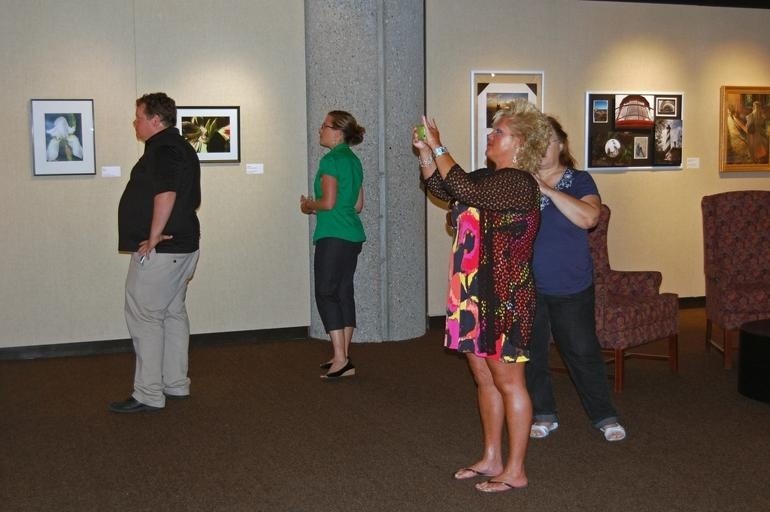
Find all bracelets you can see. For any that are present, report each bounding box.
[434,146,450,159]
[417,157,435,169]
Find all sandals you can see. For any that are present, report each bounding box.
[600,420,626,442]
[530,421,558,438]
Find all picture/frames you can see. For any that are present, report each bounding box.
[471,69,545,171]
[30,98,97,176]
[584,90,684,173]
[718,85,770,173]
[174,105,241,163]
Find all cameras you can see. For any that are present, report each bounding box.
[416,124,426,140]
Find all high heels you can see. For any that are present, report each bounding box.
[320,359,355,379]
[320,355,351,369]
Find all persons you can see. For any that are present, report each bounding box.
[108,91,202,412]
[412,98,554,492]
[525,112,627,441]
[300,109,367,380]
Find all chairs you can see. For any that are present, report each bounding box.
[701,189,769,371]
[587,203,681,393]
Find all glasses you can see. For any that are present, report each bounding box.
[491,128,514,137]
[321,124,332,129]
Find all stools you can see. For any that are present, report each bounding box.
[738,321,768,400]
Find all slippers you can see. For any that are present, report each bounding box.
[455,467,500,479]
[476,479,528,493]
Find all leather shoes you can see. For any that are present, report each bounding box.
[164,392,189,400]
[108,397,165,412]
